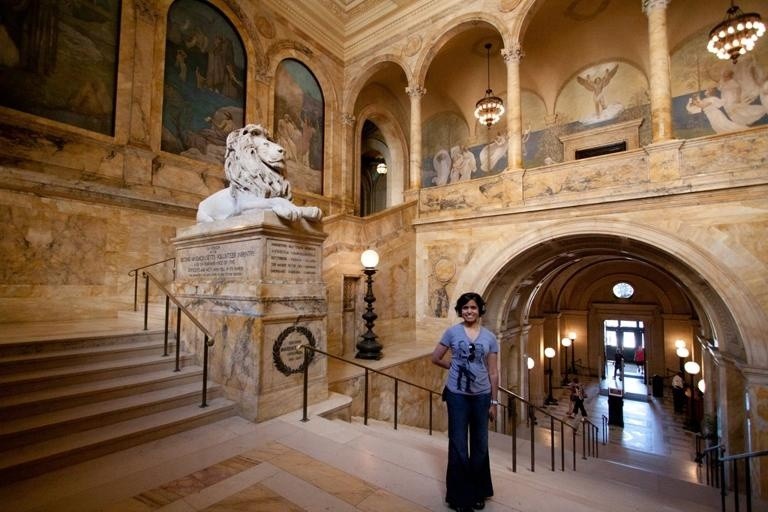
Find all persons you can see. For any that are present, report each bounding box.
[565,383,574,415]
[633,346,644,374]
[692,65,768,135]
[576,63,619,120]
[431,291,500,512]
[431,123,533,186]
[174,18,243,154]
[568,377,588,422]
[614,348,624,381]
[276,111,312,164]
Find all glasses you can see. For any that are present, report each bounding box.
[468,343,475,361]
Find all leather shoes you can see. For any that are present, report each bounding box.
[449,498,485,512]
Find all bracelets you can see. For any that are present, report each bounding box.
[490,399,498,406]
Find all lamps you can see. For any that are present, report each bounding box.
[376,162,388,174]
[704,0,766,66]
[475,42,504,130]
